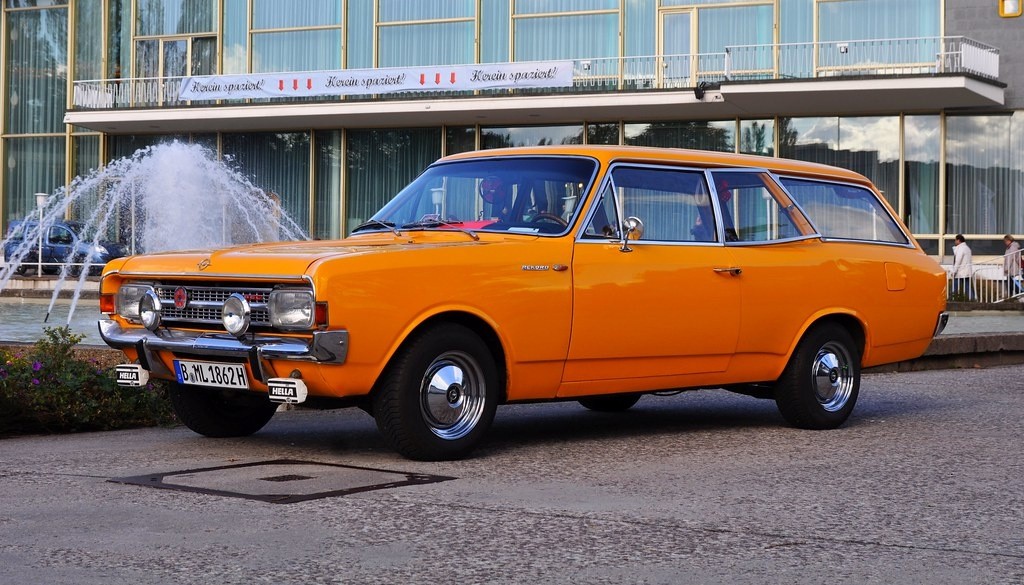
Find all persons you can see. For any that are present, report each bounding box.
[1003,234,1024,297]
[951,234,974,302]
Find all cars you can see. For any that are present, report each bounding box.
[98,146,949,461]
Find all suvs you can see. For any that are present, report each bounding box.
[3,220,123,277]
